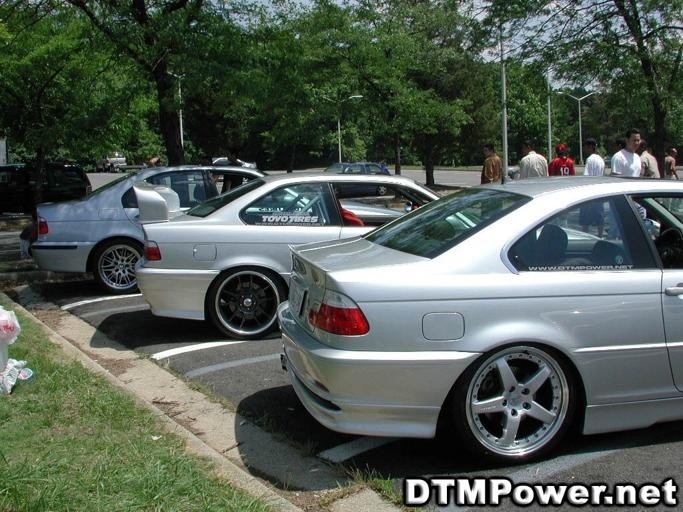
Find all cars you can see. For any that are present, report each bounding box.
[20,165,269,293]
[322,161,390,176]
[134,173,601,342]
[276,175,683,461]
[191,157,258,181]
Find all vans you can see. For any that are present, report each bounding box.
[603,156,612,168]
[0,164,92,213]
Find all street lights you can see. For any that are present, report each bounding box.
[166,71,186,150]
[556,90,602,167]
[318,93,369,163]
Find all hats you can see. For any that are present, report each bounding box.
[583,139,596,145]
[556,144,567,152]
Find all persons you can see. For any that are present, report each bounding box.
[220,144,244,195]
[664,147,678,180]
[609,127,645,237]
[146,156,167,186]
[478,140,661,216]
[194,153,219,201]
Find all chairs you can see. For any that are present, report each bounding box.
[593,241,629,265]
[535,225,593,267]
[194,183,219,202]
[336,197,365,226]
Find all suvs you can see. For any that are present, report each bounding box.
[96,152,127,172]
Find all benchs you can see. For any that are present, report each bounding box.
[117,165,144,172]
